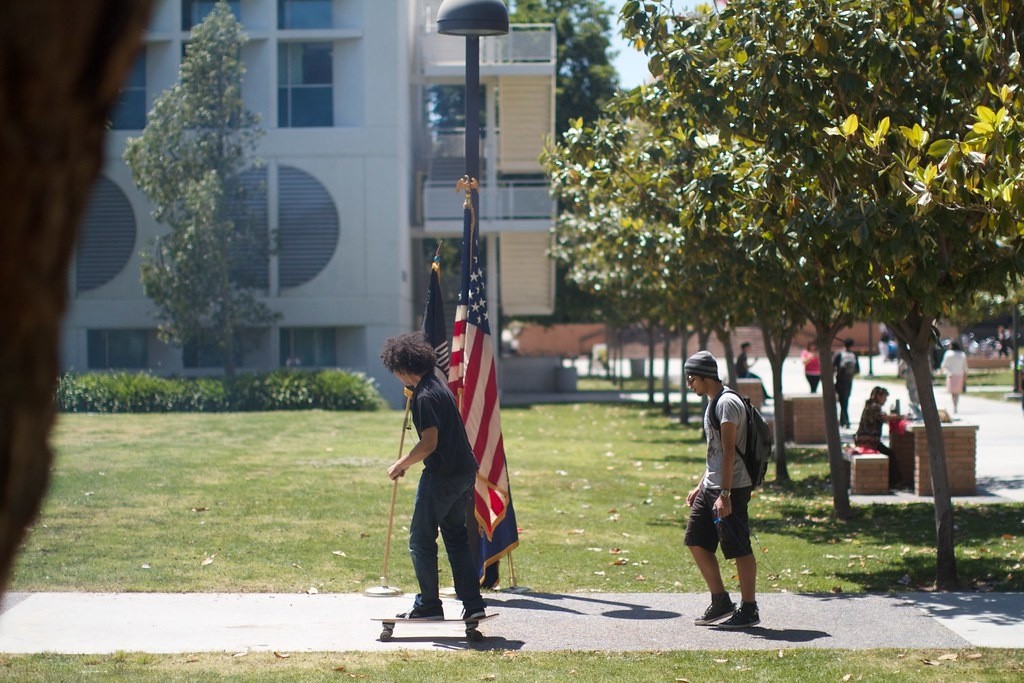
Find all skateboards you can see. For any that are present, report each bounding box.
[363,611,501,642]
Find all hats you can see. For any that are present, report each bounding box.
[685,351,719,378]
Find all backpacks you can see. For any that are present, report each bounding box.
[839,350,856,376]
[711,390,774,486]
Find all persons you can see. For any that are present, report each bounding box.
[683,349,772,629]
[736,342,772,399]
[832,337,860,429]
[898,350,934,406]
[380,330,488,621]
[940,339,968,413]
[801,341,821,393]
[857,386,903,488]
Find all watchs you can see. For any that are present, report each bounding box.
[719,488,732,498]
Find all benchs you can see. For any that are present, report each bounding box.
[841,444,889,495]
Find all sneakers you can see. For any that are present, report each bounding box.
[394,605,445,620]
[694,601,738,625]
[719,607,761,629]
[461,598,488,620]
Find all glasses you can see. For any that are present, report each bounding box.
[684,375,697,386]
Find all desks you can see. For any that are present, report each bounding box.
[722,377,763,413]
[783,392,839,445]
[886,415,980,497]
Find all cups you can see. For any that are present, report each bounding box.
[712,507,734,542]
[890,410,895,413]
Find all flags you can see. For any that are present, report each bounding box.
[403,254,450,395]
[446,186,520,586]
